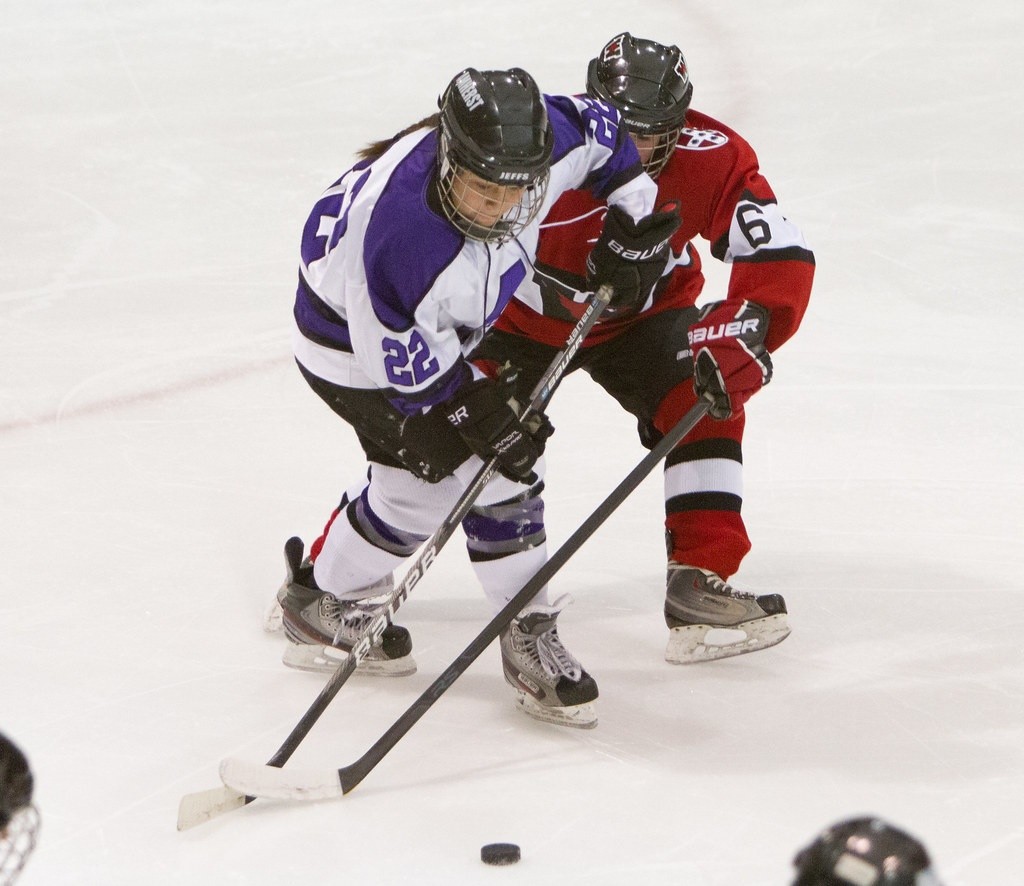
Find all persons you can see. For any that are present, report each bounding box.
[278,66,683,730]
[282,31,816,666]
[0,731,41,886]
[790,817,940,886]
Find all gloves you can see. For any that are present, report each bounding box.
[687,299,773,421]
[443,366,555,487]
[586,201,683,319]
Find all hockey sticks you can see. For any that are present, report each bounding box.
[215,396,715,805]
[144,284,616,834]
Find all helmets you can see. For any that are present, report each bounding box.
[438,67,552,247]
[791,818,930,886]
[586,32,693,180]
[1,737,41,886]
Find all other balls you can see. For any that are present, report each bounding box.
[480,843,521,867]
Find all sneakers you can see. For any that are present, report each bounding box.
[277,537,418,678]
[501,594,599,730]
[663,525,791,666]
